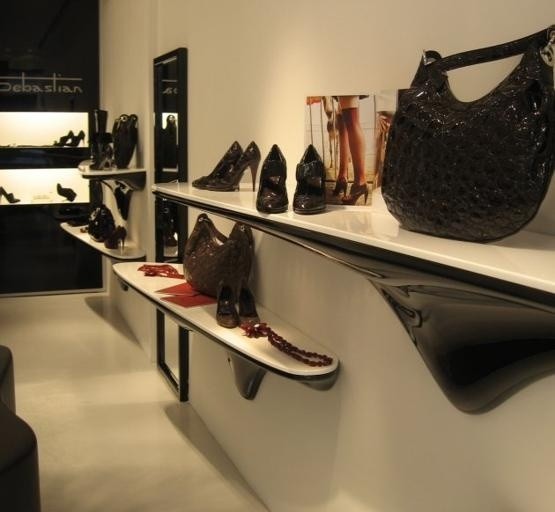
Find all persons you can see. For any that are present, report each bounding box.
[332,96,369,206]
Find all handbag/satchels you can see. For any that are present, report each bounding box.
[382,24,555,243]
[183,214,252,298]
[89,204,114,241]
[112,114,137,168]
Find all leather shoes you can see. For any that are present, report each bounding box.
[57,184,76,201]
[216,278,260,330]
[257,144,288,212]
[294,144,326,214]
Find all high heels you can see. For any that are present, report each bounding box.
[54,130,84,146]
[192,141,260,191]
[105,225,126,249]
[90,146,111,169]
[333,180,368,203]
[0,187,20,202]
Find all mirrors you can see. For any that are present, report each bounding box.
[152,46,190,403]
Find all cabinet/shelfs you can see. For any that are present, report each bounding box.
[59,220,147,294]
[149,177,472,415]
[110,261,341,402]
[77,159,147,193]
[0,92,103,290]
